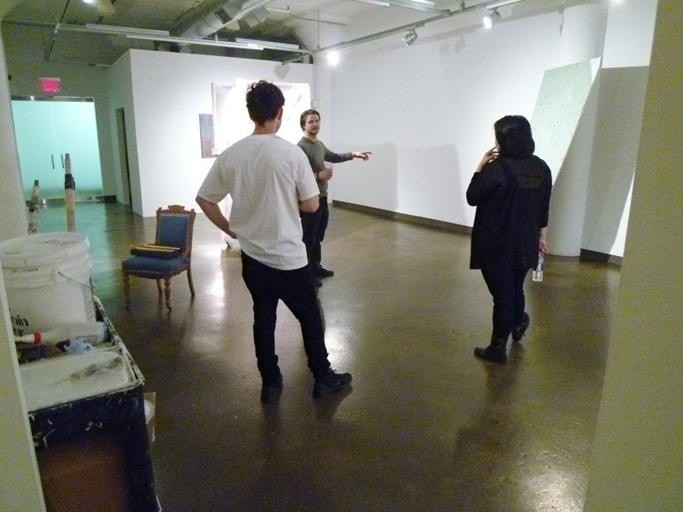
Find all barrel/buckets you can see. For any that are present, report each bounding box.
[0,233,96,336]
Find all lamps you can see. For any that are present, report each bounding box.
[54,21,319,53]
[400,6,508,49]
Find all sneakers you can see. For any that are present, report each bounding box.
[312,373,352,400]
[260,375,283,402]
[512,312,530,343]
[475,346,508,365]
[313,265,333,288]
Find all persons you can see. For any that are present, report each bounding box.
[295,109,373,288]
[194,80,352,404]
[466,114,553,365]
[32,180,40,205]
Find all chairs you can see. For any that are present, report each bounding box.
[120,202,200,314]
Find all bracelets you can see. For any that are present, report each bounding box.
[317,169,322,181]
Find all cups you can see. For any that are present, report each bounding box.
[324,163,333,170]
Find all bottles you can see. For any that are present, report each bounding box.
[531,251,543,283]
[12,320,107,352]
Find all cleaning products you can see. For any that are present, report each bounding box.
[13,322,104,347]
